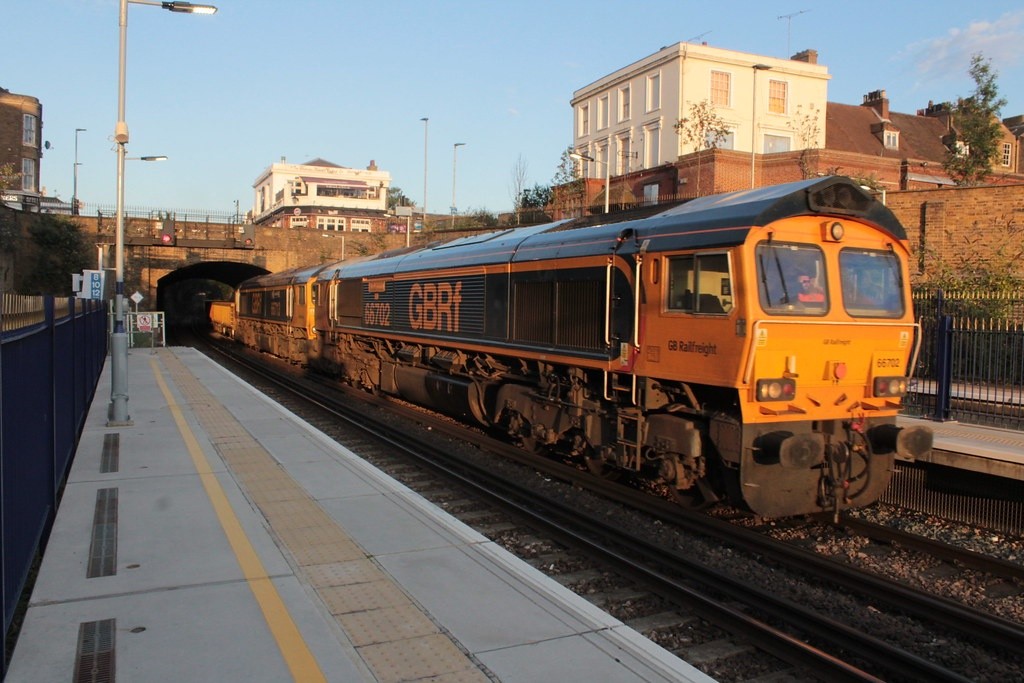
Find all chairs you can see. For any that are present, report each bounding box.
[687,293,726,313]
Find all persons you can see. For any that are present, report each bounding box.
[798,274,825,302]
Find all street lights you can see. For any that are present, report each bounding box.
[73,128,87,199]
[421,117,428,219]
[451,143,467,227]
[111,0,217,425]
[569,152,610,214]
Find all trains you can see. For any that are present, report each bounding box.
[206,174,924,525]
